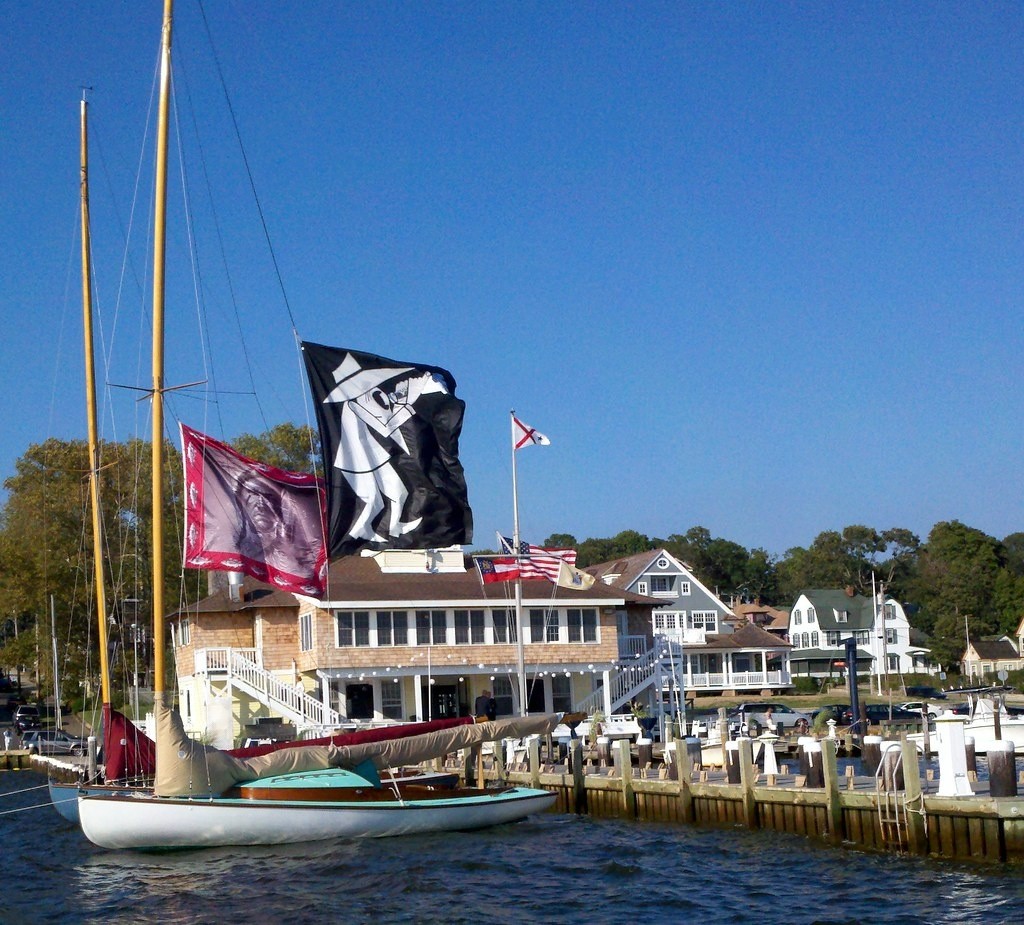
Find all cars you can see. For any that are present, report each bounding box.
[19,730,88,756]
[842,703,922,726]
[13,705,39,726]
[738,703,814,730]
[906,687,947,699]
[895,701,943,719]
[950,703,970,715]
[806,704,851,724]
[14,715,42,736]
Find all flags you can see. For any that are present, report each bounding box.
[558,560,595,590]
[178,421,328,607]
[513,416,551,449]
[476,557,520,585]
[500,536,578,585]
[299,340,473,566]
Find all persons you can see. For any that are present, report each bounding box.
[765,708,773,731]
[475,690,497,721]
[3,728,13,751]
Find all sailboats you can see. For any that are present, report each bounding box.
[74,1,588,851]
[47,88,491,824]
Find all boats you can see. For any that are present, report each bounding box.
[907,685,1024,754]
[666,719,765,768]
